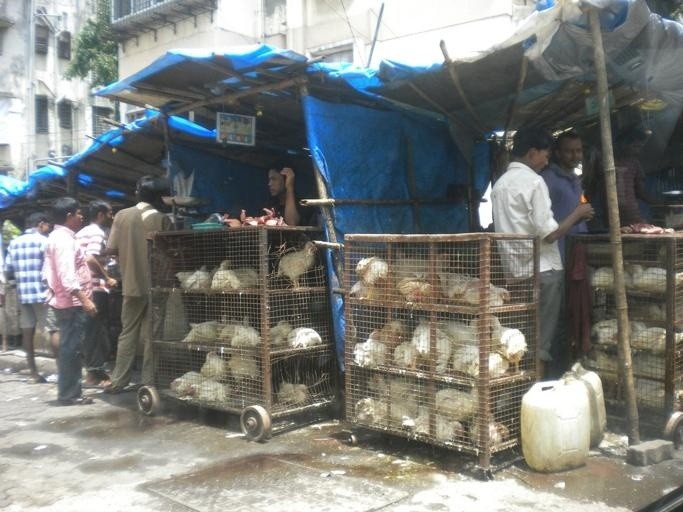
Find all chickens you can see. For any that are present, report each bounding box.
[585,259,683,413]
[168,240,323,405]
[348,252,529,449]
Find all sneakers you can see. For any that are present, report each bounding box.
[24,369,125,406]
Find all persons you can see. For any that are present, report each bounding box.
[490,127,594,379]
[262,161,317,226]
[540,130,591,351]
[0,173,164,406]
[597,123,661,228]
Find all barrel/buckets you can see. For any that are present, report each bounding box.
[521,373,591,473]
[569,362,607,448]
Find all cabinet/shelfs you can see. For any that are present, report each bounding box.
[344,232,539,465]
[579,231,683,424]
[138,227,335,418]
